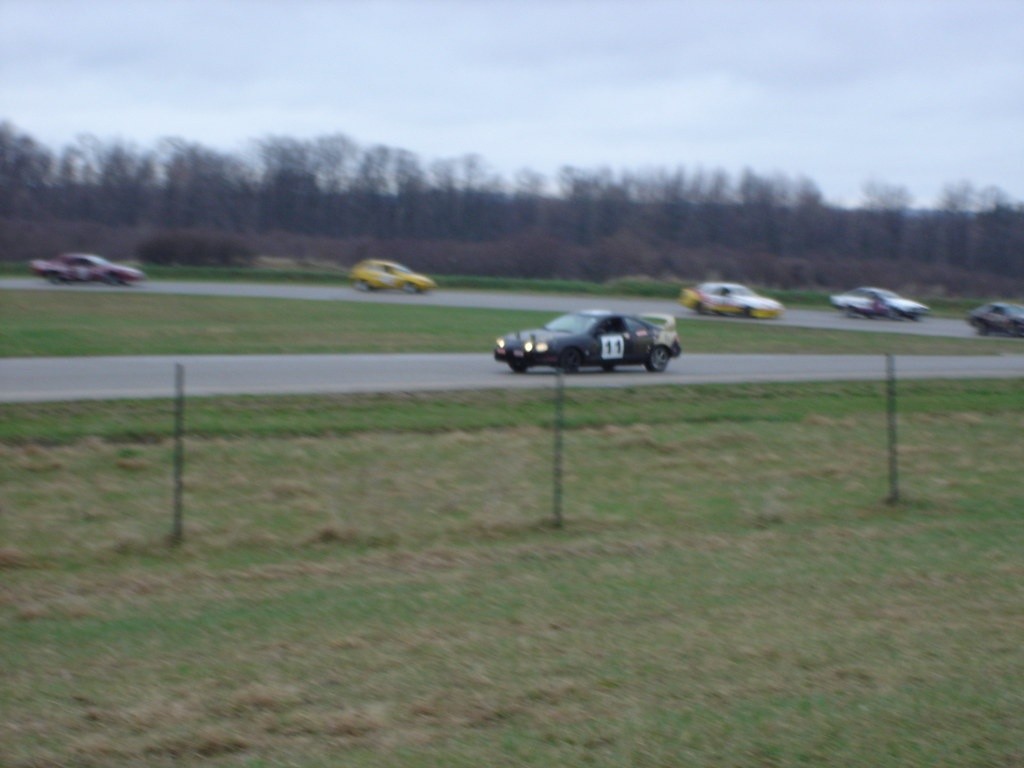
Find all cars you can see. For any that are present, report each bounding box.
[31,252,144,287]
[495,307,682,373]
[345,259,438,294]
[830,285,932,321]
[965,301,1024,338]
[680,280,786,319]
